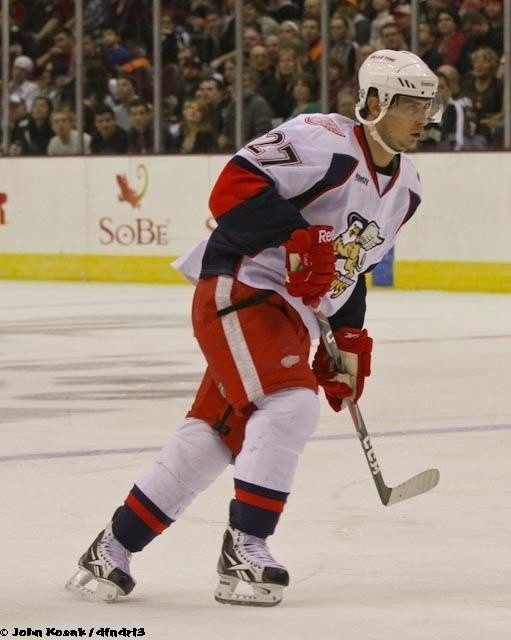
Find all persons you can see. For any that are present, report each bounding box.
[65,47,442,596]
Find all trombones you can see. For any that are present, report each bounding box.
[290,254,440,505]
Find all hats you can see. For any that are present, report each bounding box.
[14,55,34,73]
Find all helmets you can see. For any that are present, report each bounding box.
[355,49,439,125]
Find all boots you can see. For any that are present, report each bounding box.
[217,523,289,587]
[78,518,137,596]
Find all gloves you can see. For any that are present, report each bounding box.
[312,328,372,412]
[282,225,336,308]
